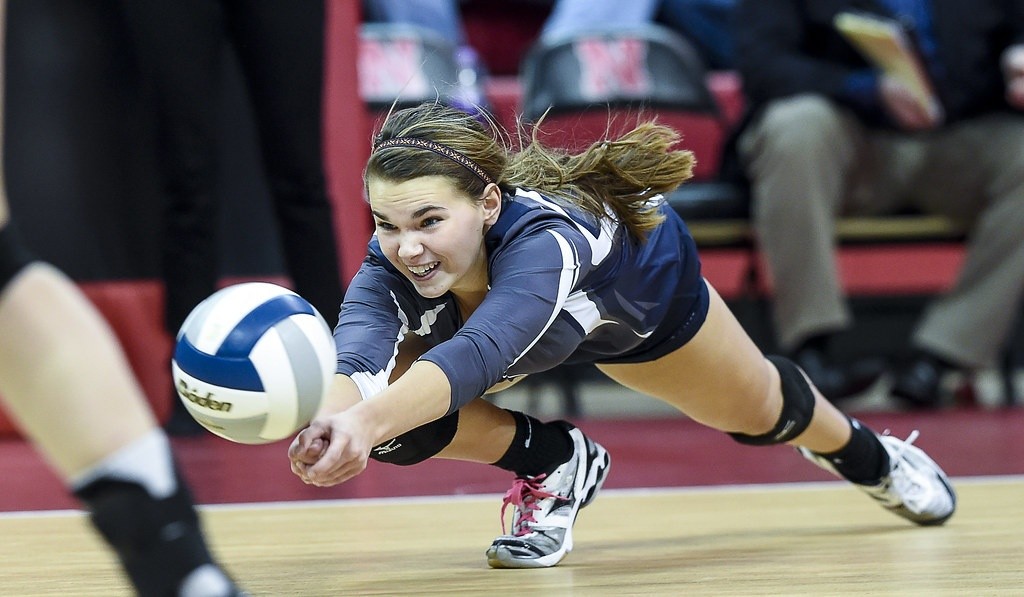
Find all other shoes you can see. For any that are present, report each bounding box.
[87,485,245,597]
[810,341,1004,415]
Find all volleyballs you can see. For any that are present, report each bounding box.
[172,282,337,445]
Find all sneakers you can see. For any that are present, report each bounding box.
[796,423,957,526]
[484,418,612,569]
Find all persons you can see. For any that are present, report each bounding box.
[289,101,957,568]
[0,188,243,597]
[726,0,1023,406]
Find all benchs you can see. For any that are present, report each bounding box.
[0,62,1024,439]
